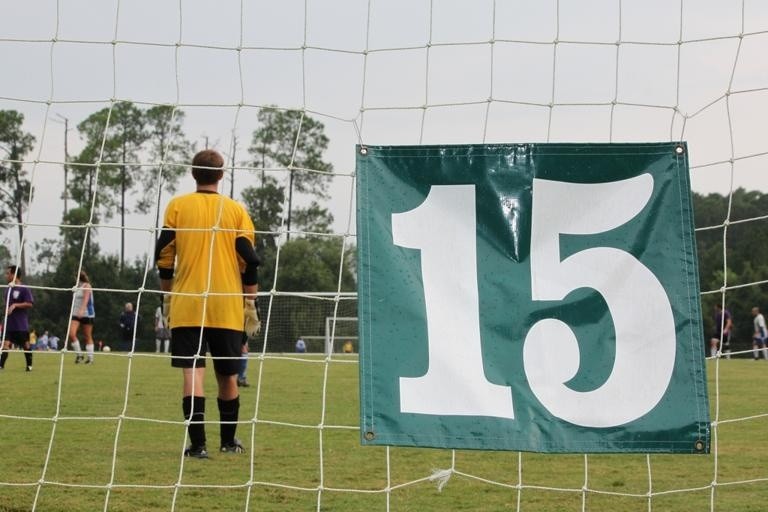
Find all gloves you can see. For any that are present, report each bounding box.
[244,297,262,341]
[159,291,172,340]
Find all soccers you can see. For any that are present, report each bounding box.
[102,345,112,352]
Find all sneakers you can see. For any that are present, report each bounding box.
[220,444,246,455]
[185,445,208,459]
[25,366,32,372]
[75,356,95,365]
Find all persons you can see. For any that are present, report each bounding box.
[340,340,353,353]
[118,302,143,353]
[48,335,60,350]
[153,148,262,460]
[41,330,49,351]
[36,335,45,351]
[234,332,261,388]
[152,304,171,355]
[294,336,306,353]
[0,263,36,371]
[68,270,96,366]
[29,328,37,350]
[710,301,733,361]
[749,305,768,362]
